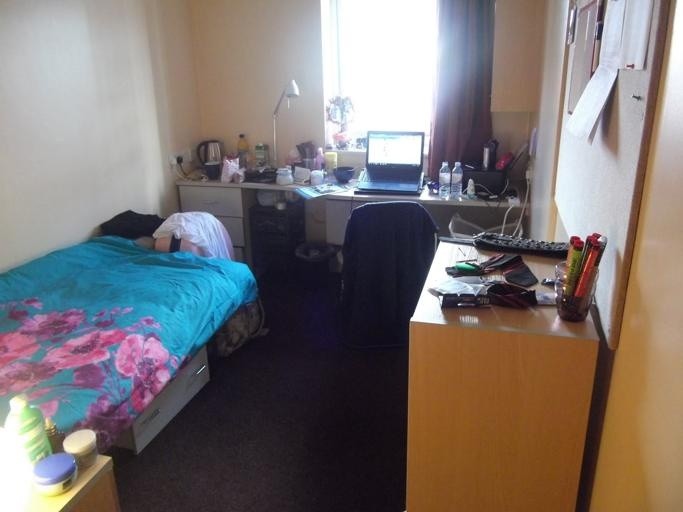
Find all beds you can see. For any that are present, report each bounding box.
[2,235,263,435]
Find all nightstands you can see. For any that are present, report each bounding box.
[34,453,123,512]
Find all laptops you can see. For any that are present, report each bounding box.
[358,131,425,193]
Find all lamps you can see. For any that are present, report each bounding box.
[273,79,301,163]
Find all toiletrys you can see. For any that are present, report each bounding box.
[45,422,62,452]
[62,429,99,468]
[34,452,79,495]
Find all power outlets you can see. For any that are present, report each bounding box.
[168,153,185,168]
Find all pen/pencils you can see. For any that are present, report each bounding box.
[560,234,608,310]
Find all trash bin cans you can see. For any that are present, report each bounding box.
[293,242,343,291]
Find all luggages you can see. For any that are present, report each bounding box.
[153,209,236,262]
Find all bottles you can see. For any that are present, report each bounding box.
[438,161,451,199]
[316,146,324,169]
[237,133,250,168]
[467,178,476,199]
[2,392,53,470]
[450,161,464,200]
[254,143,271,167]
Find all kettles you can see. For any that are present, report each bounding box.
[177,140,225,181]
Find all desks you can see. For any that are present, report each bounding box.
[175,164,530,272]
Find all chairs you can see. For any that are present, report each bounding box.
[339,201,440,353]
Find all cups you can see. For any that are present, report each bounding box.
[205,163,219,181]
[556,261,599,322]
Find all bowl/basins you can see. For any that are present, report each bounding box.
[334,166,355,183]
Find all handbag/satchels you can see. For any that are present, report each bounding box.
[215,292,265,355]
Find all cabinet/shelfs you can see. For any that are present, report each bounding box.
[405,239,599,512]
